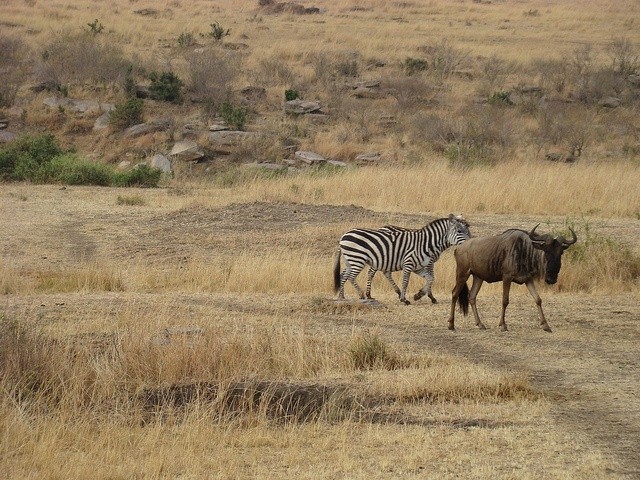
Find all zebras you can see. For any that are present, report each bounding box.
[365,213,472,304]
[333,216,469,305]
